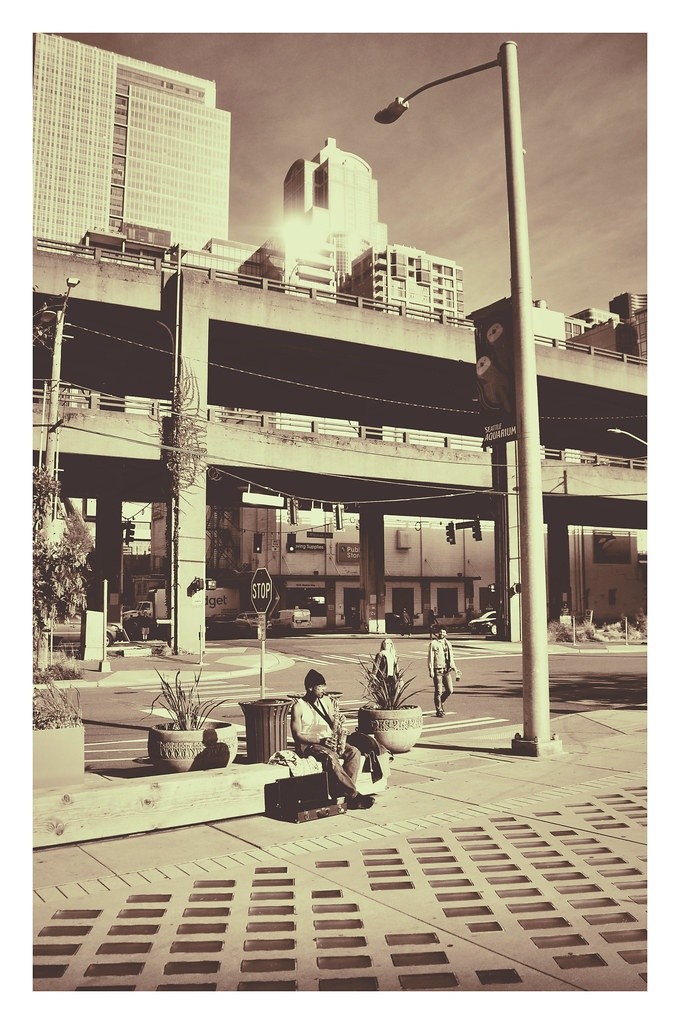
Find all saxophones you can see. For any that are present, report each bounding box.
[322,691,350,757]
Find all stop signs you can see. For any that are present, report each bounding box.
[249,567,273,613]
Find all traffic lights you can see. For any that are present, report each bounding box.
[253,533,263,554]
[445,521,456,547]
[286,533,297,554]
[124,519,135,545]
[472,520,483,542]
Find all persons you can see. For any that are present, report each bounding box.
[401,608,411,637]
[371,639,403,697]
[427,626,461,718]
[128,611,150,641]
[428,610,439,639]
[291,669,376,809]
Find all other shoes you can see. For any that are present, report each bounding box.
[344,791,375,809]
[441,703,444,711]
[436,711,444,716]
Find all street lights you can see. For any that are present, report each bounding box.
[372,41,563,759]
[38,275,77,673]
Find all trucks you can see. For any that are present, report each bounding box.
[271,608,312,634]
[121,585,242,624]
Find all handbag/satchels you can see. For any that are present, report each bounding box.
[347,727,381,755]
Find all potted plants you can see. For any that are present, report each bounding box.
[140,666,238,774]
[33,681,85,789]
[356,652,423,754]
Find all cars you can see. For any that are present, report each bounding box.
[383,613,402,622]
[468,609,498,635]
[234,610,272,631]
[49,614,123,649]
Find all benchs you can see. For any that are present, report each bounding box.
[33,750,389,850]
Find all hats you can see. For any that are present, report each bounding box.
[304,669,326,689]
